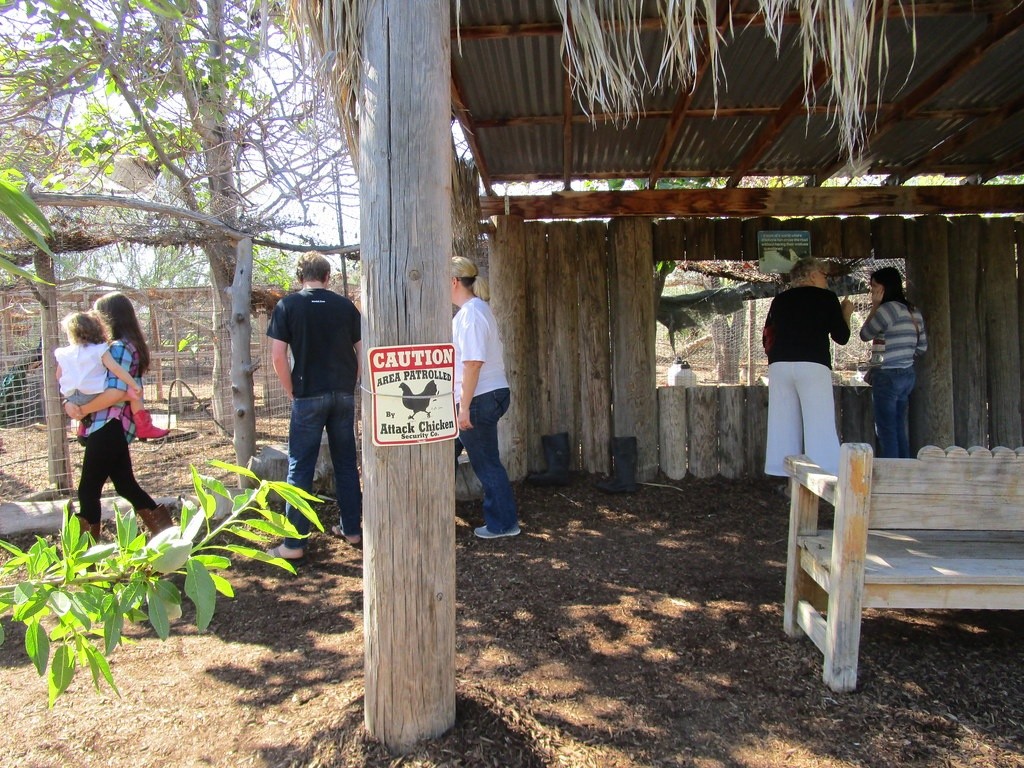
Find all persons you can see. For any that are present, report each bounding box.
[859,267,928,458]
[760,256,855,498]
[59,291,173,573]
[53,311,171,442]
[450,256,522,539]
[265,249,363,559]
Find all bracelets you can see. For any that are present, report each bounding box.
[61,399,69,407]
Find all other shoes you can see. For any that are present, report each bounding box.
[473,524,521,539]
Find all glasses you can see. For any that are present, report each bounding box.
[817,270,829,279]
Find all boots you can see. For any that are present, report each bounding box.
[75,513,100,548]
[131,410,172,442]
[137,502,173,535]
[537,431,572,486]
[76,421,88,447]
[594,436,639,493]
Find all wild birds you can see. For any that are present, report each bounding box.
[0,336,43,430]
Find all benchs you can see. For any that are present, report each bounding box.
[783,443,1024,693]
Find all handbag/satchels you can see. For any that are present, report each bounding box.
[863,367,873,387]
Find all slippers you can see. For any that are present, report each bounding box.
[336,525,361,544]
[265,544,304,560]
